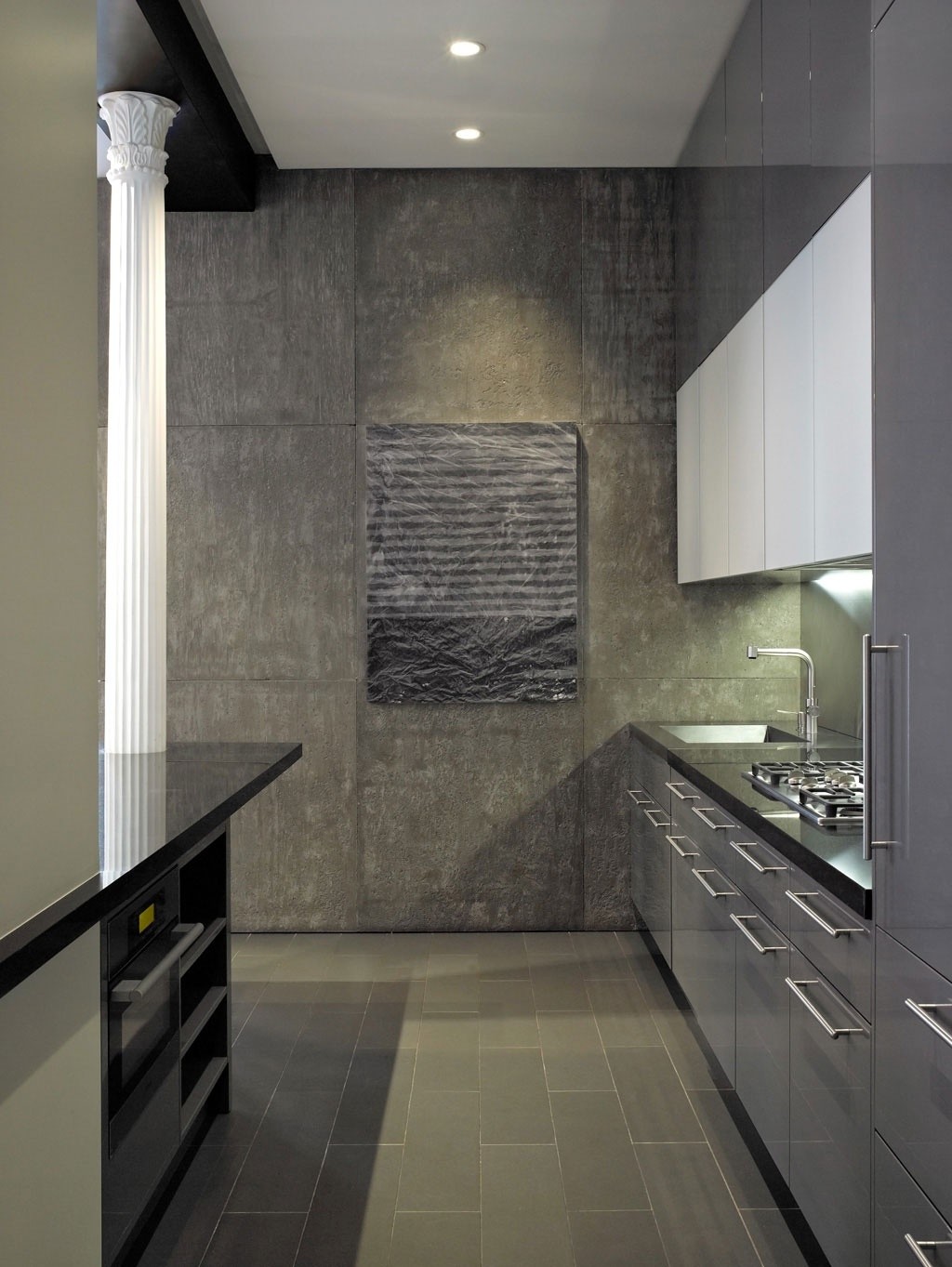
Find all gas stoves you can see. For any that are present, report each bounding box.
[743,756,865,830]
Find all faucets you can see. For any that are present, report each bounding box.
[746,645,822,735]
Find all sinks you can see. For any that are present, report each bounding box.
[658,723,810,744]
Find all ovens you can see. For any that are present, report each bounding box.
[100,862,184,1267]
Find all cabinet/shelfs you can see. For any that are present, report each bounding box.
[766,175,874,572]
[733,824,875,1267]
[669,767,736,1092]
[625,736,673,970]
[672,293,767,583]
[870,924,950,1267]
